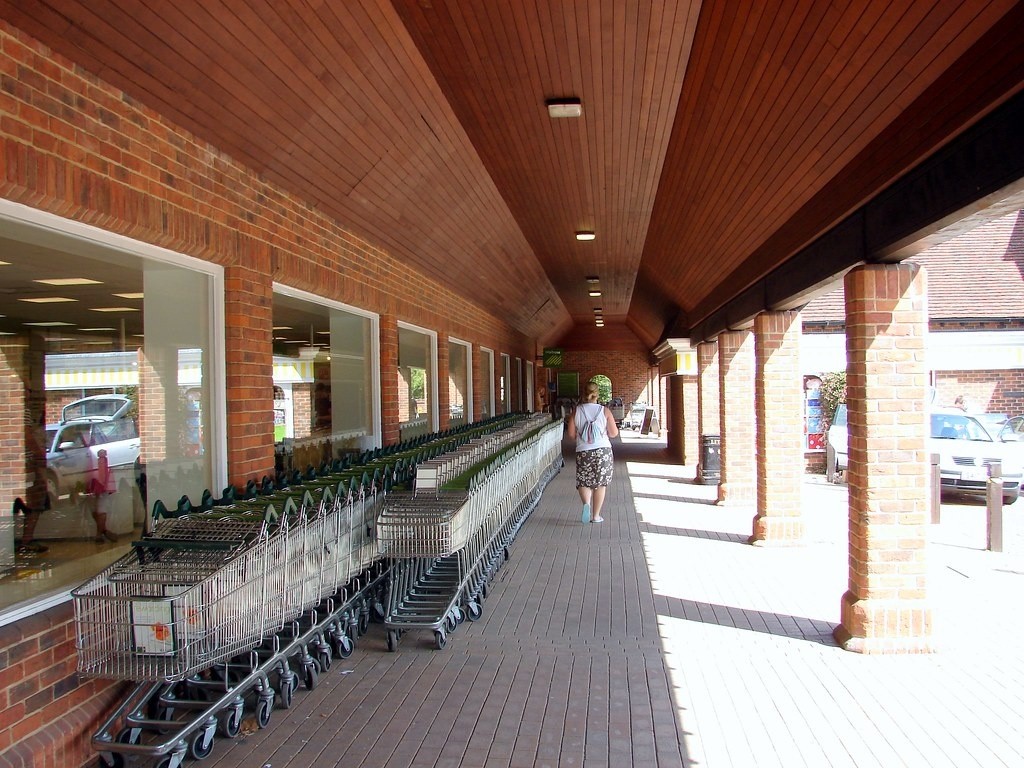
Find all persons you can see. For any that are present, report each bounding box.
[411,395,417,420]
[535,387,546,412]
[80,428,120,545]
[313,383,332,427]
[5,374,52,552]
[568,382,618,523]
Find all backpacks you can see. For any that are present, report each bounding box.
[578,403,607,444]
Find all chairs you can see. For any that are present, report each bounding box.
[941,427,959,438]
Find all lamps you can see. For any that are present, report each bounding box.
[589,290,602,297]
[585,276,601,284]
[546,96,582,118]
[575,230,595,241]
[593,308,604,312]
[594,314,605,327]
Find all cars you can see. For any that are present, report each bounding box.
[44,394,145,499]
[829,403,1024,505]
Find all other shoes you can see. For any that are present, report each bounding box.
[20,544,48,554]
[95,538,103,544]
[104,530,117,543]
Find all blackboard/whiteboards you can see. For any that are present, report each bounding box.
[640,408,653,435]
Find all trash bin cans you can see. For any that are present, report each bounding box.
[699,433,720,486]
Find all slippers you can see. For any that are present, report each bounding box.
[581,504,590,524]
[592,515,605,523]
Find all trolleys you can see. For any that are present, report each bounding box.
[71,411,564,768]
